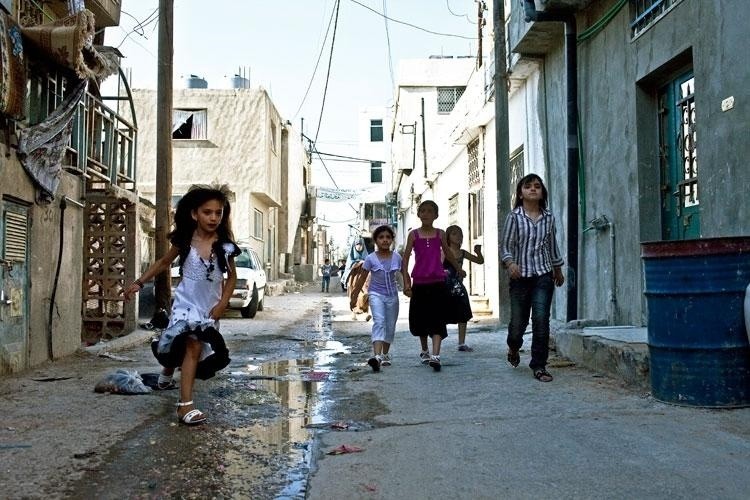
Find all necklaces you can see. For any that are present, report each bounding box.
[188,242,220,281]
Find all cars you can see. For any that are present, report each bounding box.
[151,245,267,319]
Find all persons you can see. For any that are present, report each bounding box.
[353,225,413,370]
[123,189,242,425]
[337,260,349,291]
[320,259,332,292]
[341,238,372,321]
[500,173,565,382]
[439,225,484,352]
[402,199,466,372]
[331,263,338,276]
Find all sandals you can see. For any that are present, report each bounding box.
[534,369,553,382]
[420,349,429,364]
[458,344,473,352]
[508,353,520,367]
[158,373,173,389]
[175,399,206,425]
[429,354,441,371]
[382,354,391,366]
[368,354,382,371]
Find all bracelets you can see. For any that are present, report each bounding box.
[134,279,144,287]
